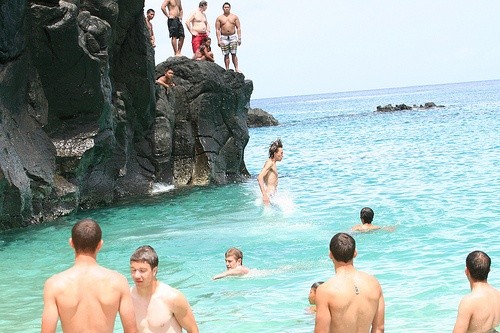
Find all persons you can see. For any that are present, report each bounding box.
[192,37,214,62]
[314,232,385,333]
[215,2,241,72]
[212,247,248,280]
[452,250,500,333]
[155,68,177,88]
[129,245,199,333]
[146,8,156,51]
[40,219,137,333]
[351,207,395,233]
[305,281,326,313]
[256,138,284,205]
[186,0,210,54]
[161,0,185,57]
